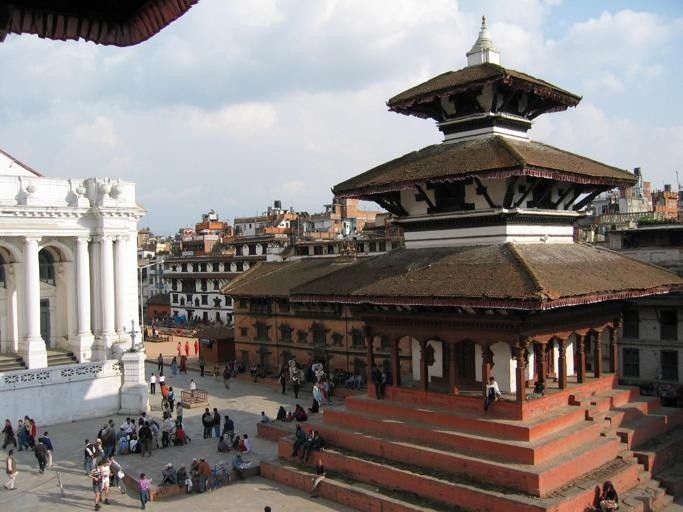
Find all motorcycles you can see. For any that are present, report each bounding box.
[161,432,175,448]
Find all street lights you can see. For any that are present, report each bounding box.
[138,259,165,342]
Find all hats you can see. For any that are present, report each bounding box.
[166,463,173,468]
[120,437,126,442]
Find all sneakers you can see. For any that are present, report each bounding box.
[94,499,112,511]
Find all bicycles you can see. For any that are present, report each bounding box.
[115,471,126,495]
[202,460,229,493]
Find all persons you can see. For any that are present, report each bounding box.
[483,376,501,414]
[532,378,546,395]
[599,480,619,511]
